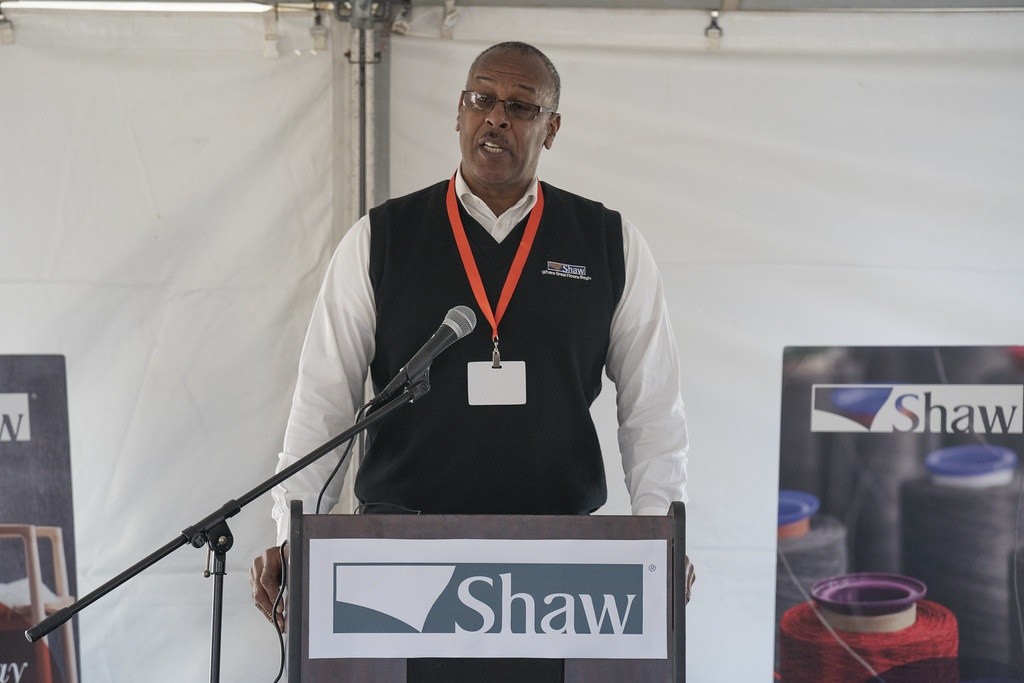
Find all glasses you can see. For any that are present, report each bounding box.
[461,91,555,121]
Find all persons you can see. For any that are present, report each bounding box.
[248,41,696,636]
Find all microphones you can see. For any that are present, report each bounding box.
[371,303,477,405]
